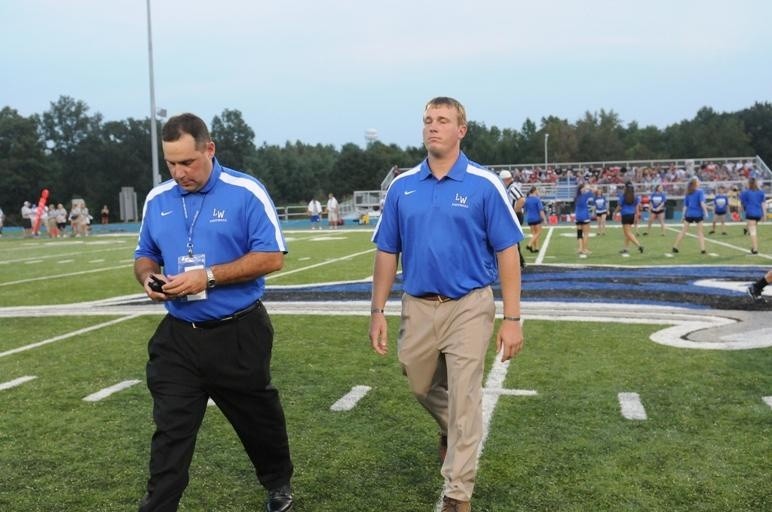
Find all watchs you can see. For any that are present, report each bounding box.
[203,266,217,289]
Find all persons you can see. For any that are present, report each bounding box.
[379,194,386,216]
[307,196,323,231]
[0,208,5,236]
[322,192,339,230]
[393,162,401,178]
[20,197,93,240]
[101,205,110,224]
[746,270,771,305]
[136,112,296,512]
[368,96,525,511]
[498,159,769,273]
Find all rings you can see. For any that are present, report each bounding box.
[181,291,186,296]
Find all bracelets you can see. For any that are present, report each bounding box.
[369,308,384,315]
[503,316,520,322]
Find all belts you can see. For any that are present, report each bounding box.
[420,294,450,303]
[192,297,263,329]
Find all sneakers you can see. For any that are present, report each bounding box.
[440,494,471,511]
[746,283,768,306]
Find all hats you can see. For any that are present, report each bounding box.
[499,170,513,179]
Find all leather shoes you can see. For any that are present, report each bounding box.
[266,478,293,512]
